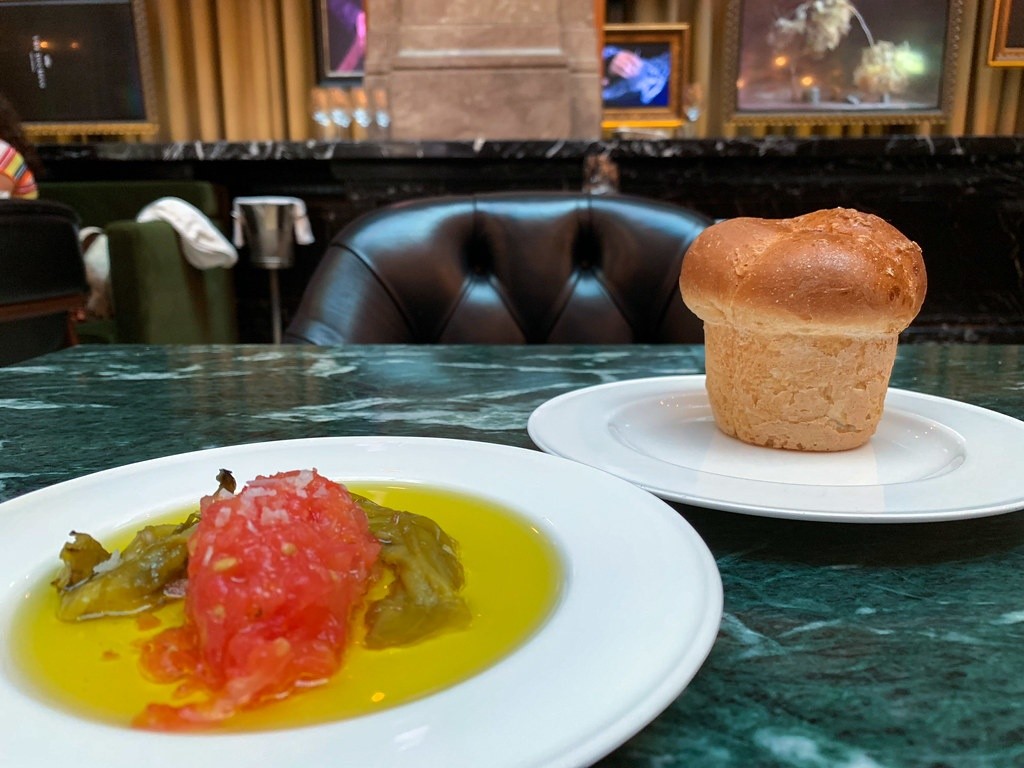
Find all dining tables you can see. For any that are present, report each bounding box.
[0,345,1024,768]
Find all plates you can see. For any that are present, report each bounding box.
[0,436,722,768]
[527,377,1024,523]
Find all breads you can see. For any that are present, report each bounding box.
[677,207,926,452]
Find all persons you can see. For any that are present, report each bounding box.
[0,88,39,200]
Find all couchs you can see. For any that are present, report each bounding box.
[31,182,239,347]
[284,189,714,345]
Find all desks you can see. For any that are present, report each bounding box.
[0,290,91,368]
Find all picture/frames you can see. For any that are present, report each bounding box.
[985,0,1024,69]
[709,0,965,128]
[0,0,160,136]
[315,1,365,81]
[600,20,689,129]
